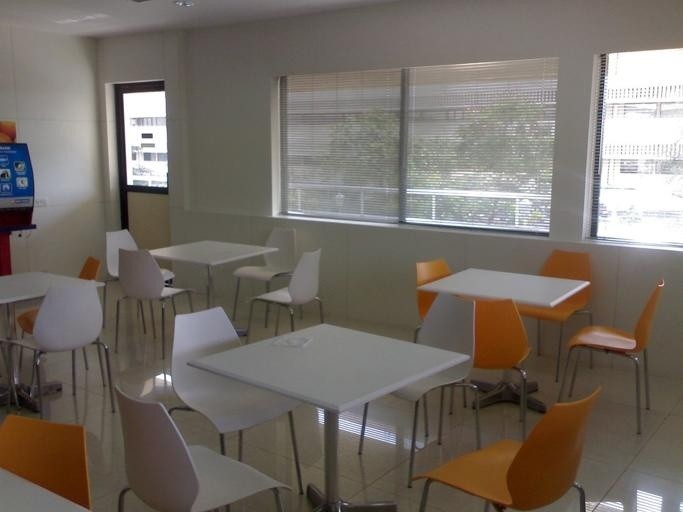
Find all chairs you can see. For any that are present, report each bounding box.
[1,224,106,511]
